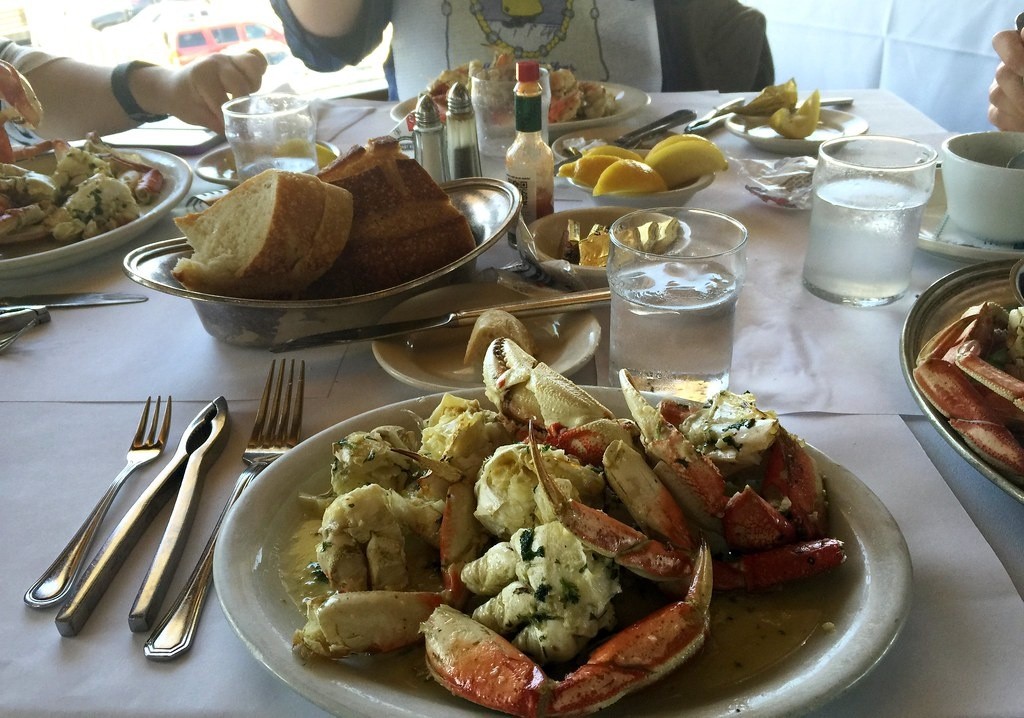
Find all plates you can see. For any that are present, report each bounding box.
[908,165,1024,262]
[192,135,342,189]
[0,144,193,279]
[724,102,869,156]
[372,284,602,393]
[900,257,1024,508]
[389,78,652,136]
[123,177,523,349]
[212,385,914,717]
[521,204,692,290]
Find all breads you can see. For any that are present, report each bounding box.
[464,307,542,365]
[174,135,476,299]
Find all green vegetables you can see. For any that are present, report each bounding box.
[307,415,756,654]
[52,151,105,219]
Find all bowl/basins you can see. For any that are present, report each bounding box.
[940,130,1024,245]
[568,146,717,208]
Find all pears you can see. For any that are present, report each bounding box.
[770,89,821,137]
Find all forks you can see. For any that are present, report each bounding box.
[0,318,38,352]
[142,356,309,661]
[185,190,235,209]
[21,395,173,609]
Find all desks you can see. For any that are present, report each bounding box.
[0,83,1024,718]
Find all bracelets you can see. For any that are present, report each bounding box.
[111,60,168,123]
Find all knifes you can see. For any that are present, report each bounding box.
[268,287,615,358]
[0,293,148,306]
[552,107,697,179]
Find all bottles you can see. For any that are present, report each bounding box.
[415,82,483,187]
[503,60,554,249]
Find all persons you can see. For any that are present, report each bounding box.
[269,0,775,103]
[987,11,1024,132]
[0,35,268,143]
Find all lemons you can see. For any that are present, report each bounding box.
[556,134,729,197]
[277,138,337,169]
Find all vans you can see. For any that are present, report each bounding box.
[175,20,288,66]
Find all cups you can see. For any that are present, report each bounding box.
[606,206,747,401]
[470,67,551,159]
[220,95,318,189]
[800,136,936,309]
